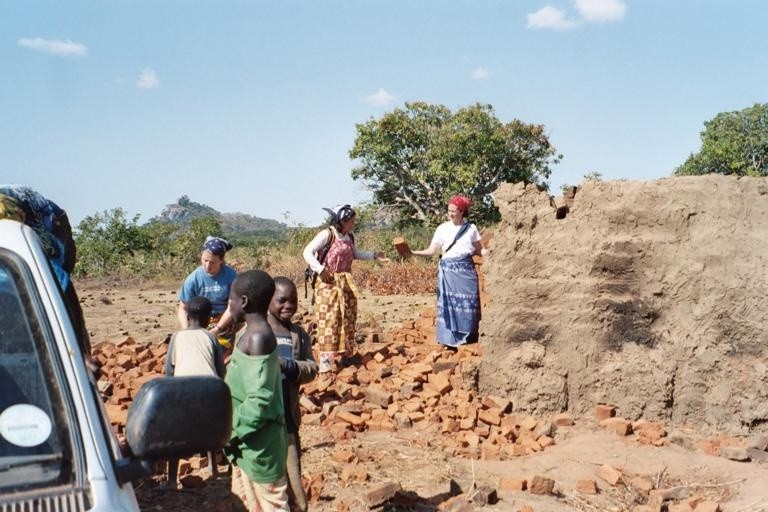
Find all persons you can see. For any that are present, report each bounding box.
[412,195,483,351]
[177,234,246,379]
[154,296,227,492]
[222,269,292,512]
[302,204,390,374]
[267,275,320,512]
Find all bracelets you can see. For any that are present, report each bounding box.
[214,324,221,330]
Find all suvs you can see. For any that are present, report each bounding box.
[0,214,232,511]
